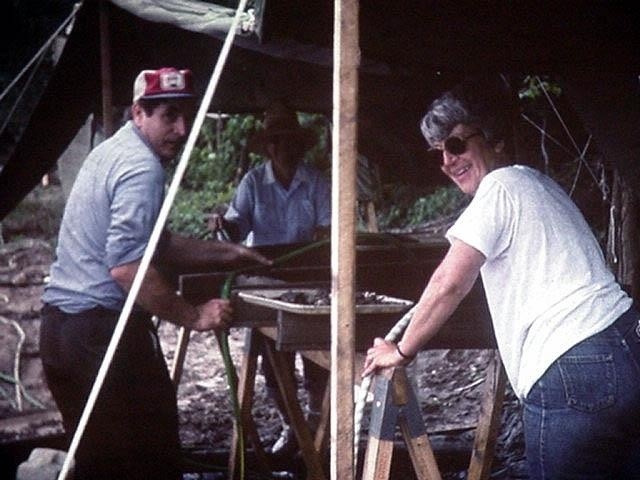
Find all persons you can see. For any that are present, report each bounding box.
[209,106,331,455]
[362,84,640,478]
[40,69,274,479]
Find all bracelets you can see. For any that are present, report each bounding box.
[397,346,414,361]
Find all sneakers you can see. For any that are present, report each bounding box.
[271,424,296,454]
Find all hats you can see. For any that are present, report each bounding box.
[249,109,321,155]
[132,68,198,104]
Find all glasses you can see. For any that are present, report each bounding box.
[424,132,484,165]
